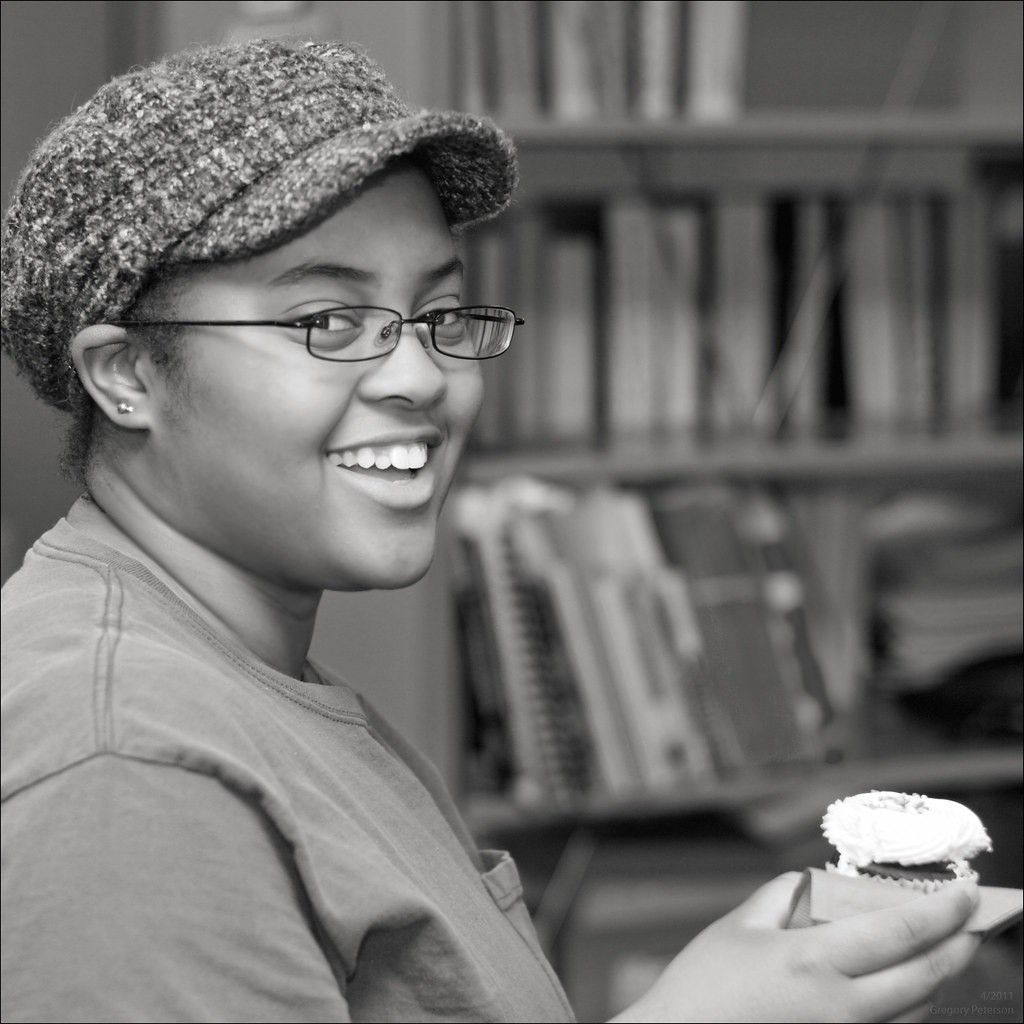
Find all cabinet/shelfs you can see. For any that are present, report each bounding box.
[148,1,1024,844]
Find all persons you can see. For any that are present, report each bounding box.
[1,38,982,1023]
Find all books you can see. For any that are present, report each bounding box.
[440,1,1023,801]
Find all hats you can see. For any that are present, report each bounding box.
[0,34,520,412]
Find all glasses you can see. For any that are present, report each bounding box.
[106,305,525,362]
